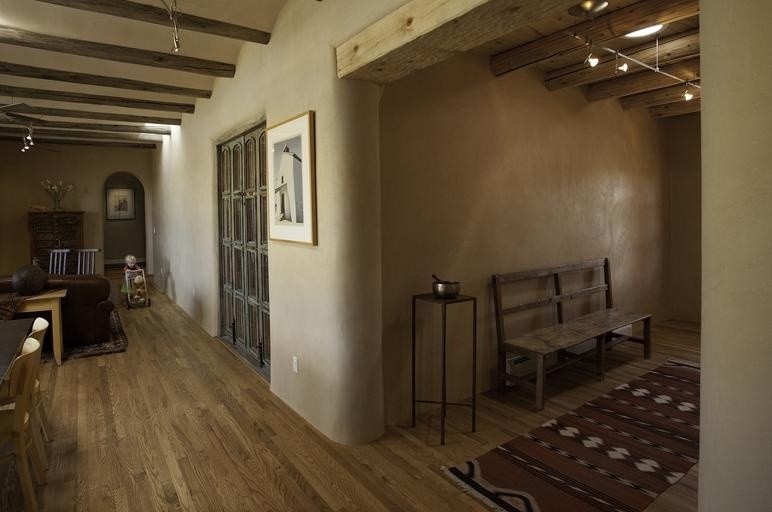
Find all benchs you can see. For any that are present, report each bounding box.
[489,257,652,412]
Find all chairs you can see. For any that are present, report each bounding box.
[74,248,101,275]
[0,317,55,473]
[0,338,49,512]
[45,248,71,275]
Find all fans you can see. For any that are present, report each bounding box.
[0,101,48,126]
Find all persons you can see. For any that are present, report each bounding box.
[121,253,142,309]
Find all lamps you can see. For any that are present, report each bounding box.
[572,33,701,103]
[158,0,184,55]
[20,122,35,154]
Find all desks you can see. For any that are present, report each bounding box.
[0,288,68,367]
[410,292,478,447]
[0,318,35,388]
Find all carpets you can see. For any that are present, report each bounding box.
[439,357,700,512]
[40,310,128,364]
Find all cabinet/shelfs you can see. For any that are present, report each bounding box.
[27,210,86,275]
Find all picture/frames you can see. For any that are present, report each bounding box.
[265,109,318,246]
[105,187,136,221]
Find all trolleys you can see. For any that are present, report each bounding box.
[123,267,152,308]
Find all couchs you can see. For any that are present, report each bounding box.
[0,276,115,347]
